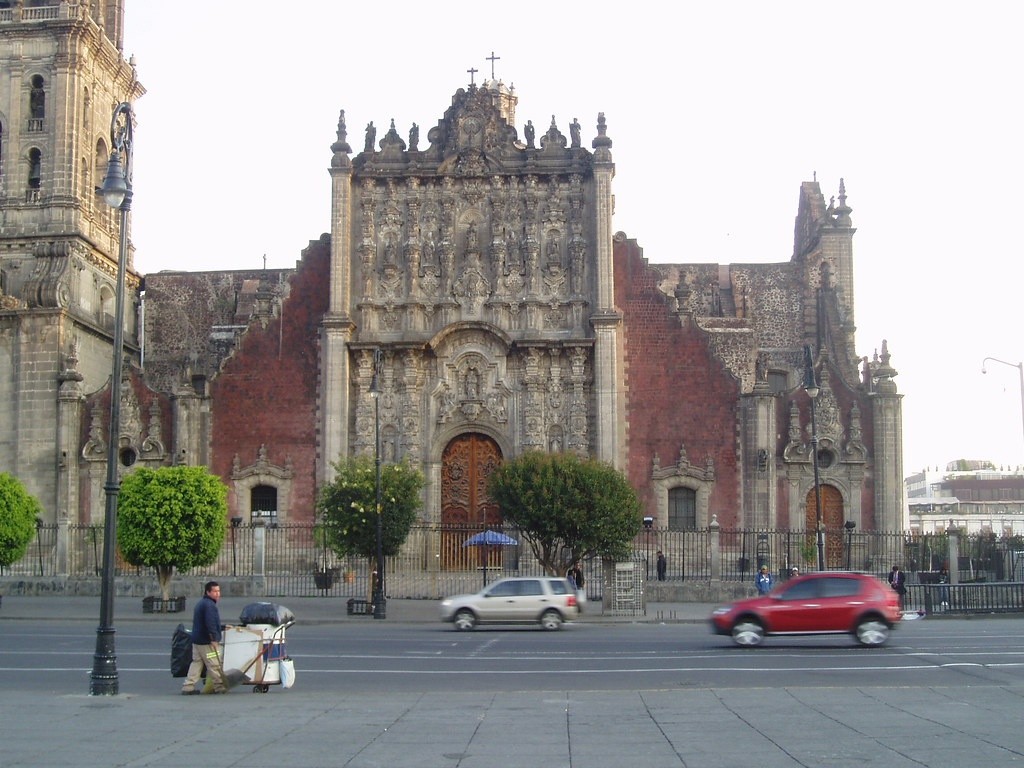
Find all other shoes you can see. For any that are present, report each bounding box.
[182,689,201,695]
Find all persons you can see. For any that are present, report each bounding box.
[182,582,233,695]
[790,567,799,578]
[937,567,948,605]
[888,566,907,611]
[755,565,773,597]
[568,563,585,612]
[657,551,667,581]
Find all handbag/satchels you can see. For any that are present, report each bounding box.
[280,659,296,688]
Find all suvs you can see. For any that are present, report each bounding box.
[440,577,577,633]
[710,570,903,648]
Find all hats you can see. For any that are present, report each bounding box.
[761,565,767,570]
[793,567,798,571]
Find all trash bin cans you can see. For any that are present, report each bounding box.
[757,554,769,572]
[779,568,792,581]
[739,557,750,572]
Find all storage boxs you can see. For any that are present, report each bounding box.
[224,624,285,684]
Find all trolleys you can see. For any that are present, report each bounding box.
[201,620,298,693]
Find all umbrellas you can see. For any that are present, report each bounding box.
[462,529,517,547]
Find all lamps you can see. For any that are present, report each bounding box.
[758,448,768,473]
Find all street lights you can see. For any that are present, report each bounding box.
[980,357,1024,437]
[803,341,826,570]
[367,350,388,619]
[88,102,135,694]
